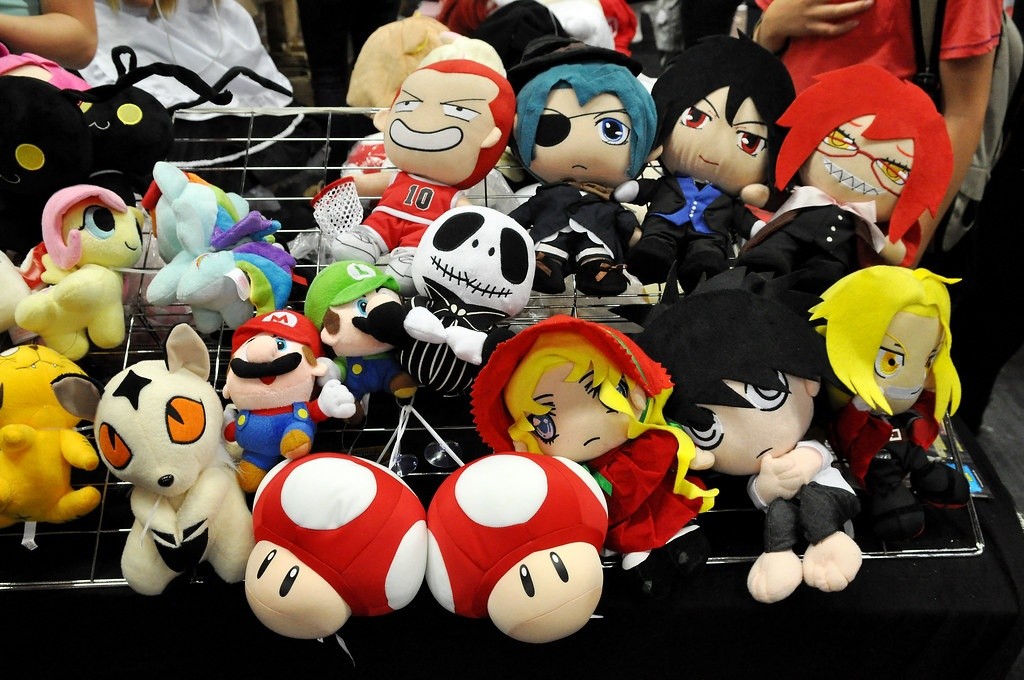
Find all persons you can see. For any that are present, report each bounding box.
[744,0,1006,269]
[298,0,403,108]
[0,2,99,82]
[77,1,296,121]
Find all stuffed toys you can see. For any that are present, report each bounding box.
[351,202,537,400]
[222,310,357,494]
[304,259,417,405]
[635,289,864,607]
[470,314,715,585]
[242,451,426,641]
[614,30,797,307]
[428,452,610,644]
[142,160,297,335]
[504,33,658,296]
[0,344,102,531]
[328,59,516,300]
[46,322,253,595]
[1,75,92,258]
[806,264,970,541]
[15,185,140,361]
[734,63,954,297]
[80,44,294,210]
